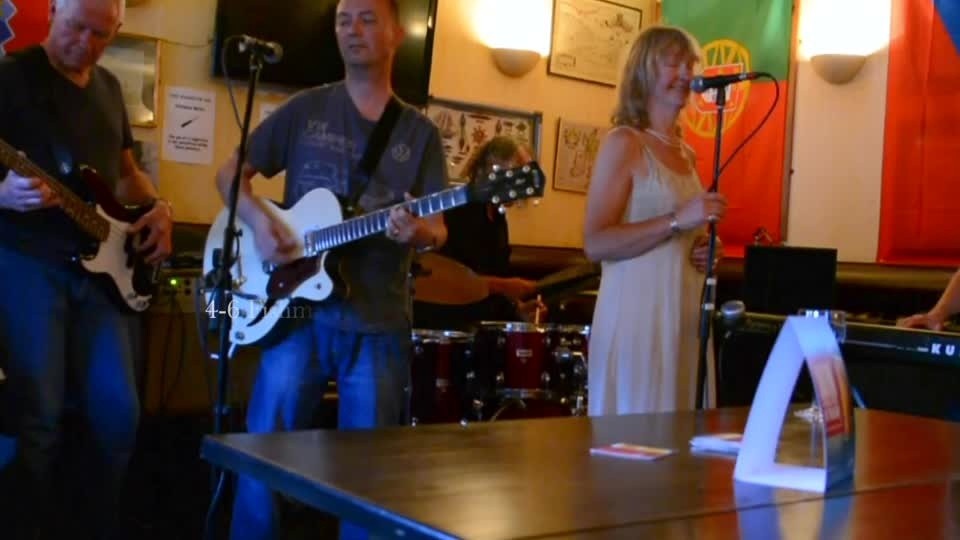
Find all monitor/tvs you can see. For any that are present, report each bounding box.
[209,1,439,109]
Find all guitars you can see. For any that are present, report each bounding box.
[0,137,159,311]
[203,158,547,348]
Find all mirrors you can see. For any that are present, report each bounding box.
[99,34,166,128]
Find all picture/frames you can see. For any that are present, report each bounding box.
[554,116,617,193]
[547,0,642,88]
[426,97,545,188]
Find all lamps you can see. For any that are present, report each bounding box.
[492,46,541,78]
[808,53,868,83]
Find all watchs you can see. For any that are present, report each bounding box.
[414,240,435,255]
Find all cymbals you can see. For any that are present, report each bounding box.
[408,251,487,305]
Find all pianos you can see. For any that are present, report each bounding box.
[713,308,960,414]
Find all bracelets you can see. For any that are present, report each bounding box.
[149,196,175,220]
[669,211,679,234]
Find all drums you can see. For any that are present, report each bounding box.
[548,324,595,397]
[481,319,550,403]
[408,329,472,427]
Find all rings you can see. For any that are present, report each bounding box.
[708,216,713,223]
[393,229,400,236]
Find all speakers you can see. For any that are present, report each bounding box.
[144,268,256,409]
[743,244,838,318]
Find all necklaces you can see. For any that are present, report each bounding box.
[644,127,684,148]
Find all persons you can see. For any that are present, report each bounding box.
[895,264,960,334]
[0,0,174,540]
[583,26,726,417]
[219,0,449,540]
[413,137,548,331]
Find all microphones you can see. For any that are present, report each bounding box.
[238,34,283,64]
[688,70,762,95]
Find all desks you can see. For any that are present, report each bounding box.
[542,484,960,540]
[200,403,960,540]
